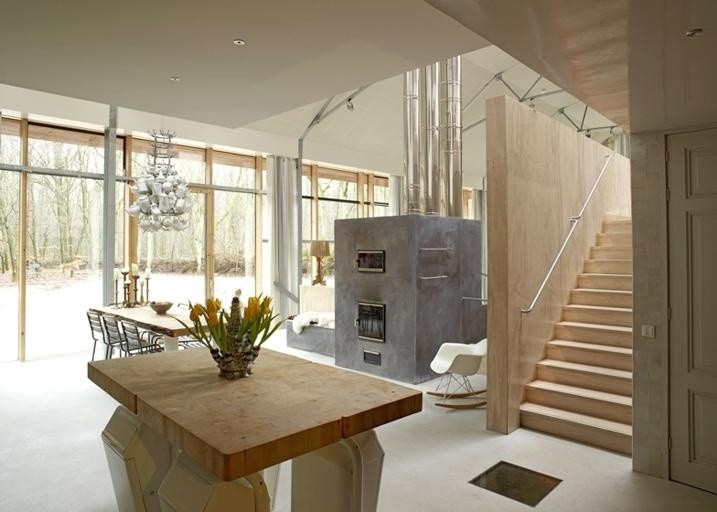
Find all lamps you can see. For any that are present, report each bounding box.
[310,240,331,285]
[126,125,194,232]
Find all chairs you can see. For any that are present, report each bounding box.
[86,312,165,361]
[425,338,488,409]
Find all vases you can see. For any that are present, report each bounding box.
[210,349,259,379]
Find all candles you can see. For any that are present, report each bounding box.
[115,264,151,283]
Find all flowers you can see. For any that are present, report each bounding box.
[167,292,293,356]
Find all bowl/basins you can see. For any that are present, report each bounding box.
[149,302,173,315]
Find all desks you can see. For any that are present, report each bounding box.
[87,347,423,510]
[86,302,215,349]
